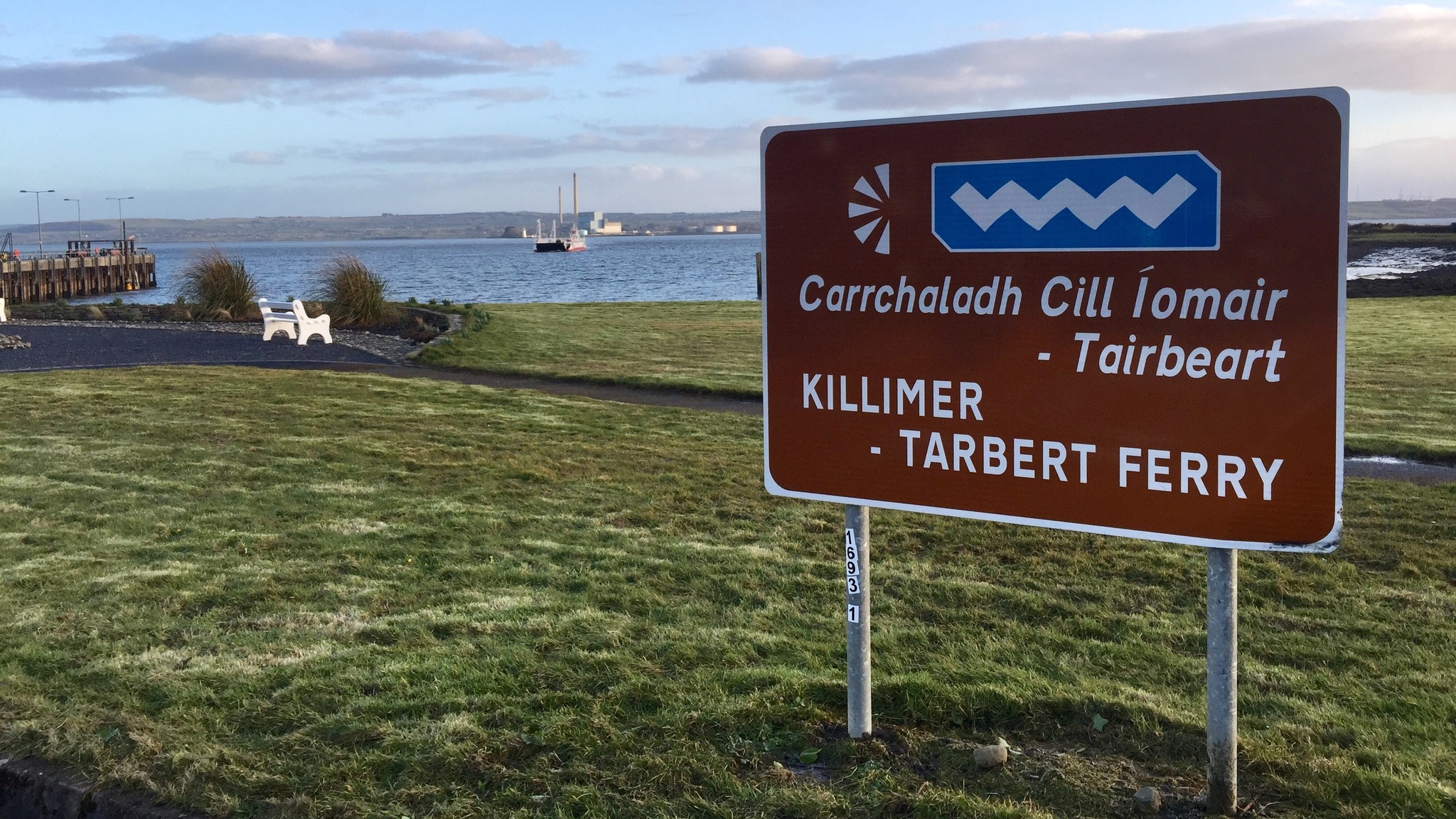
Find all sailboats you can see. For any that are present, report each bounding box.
[534,218,587,254]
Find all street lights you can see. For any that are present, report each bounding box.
[20,190,57,259]
[64,199,82,240]
[106,196,134,240]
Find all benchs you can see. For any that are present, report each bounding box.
[257,297,333,344]
